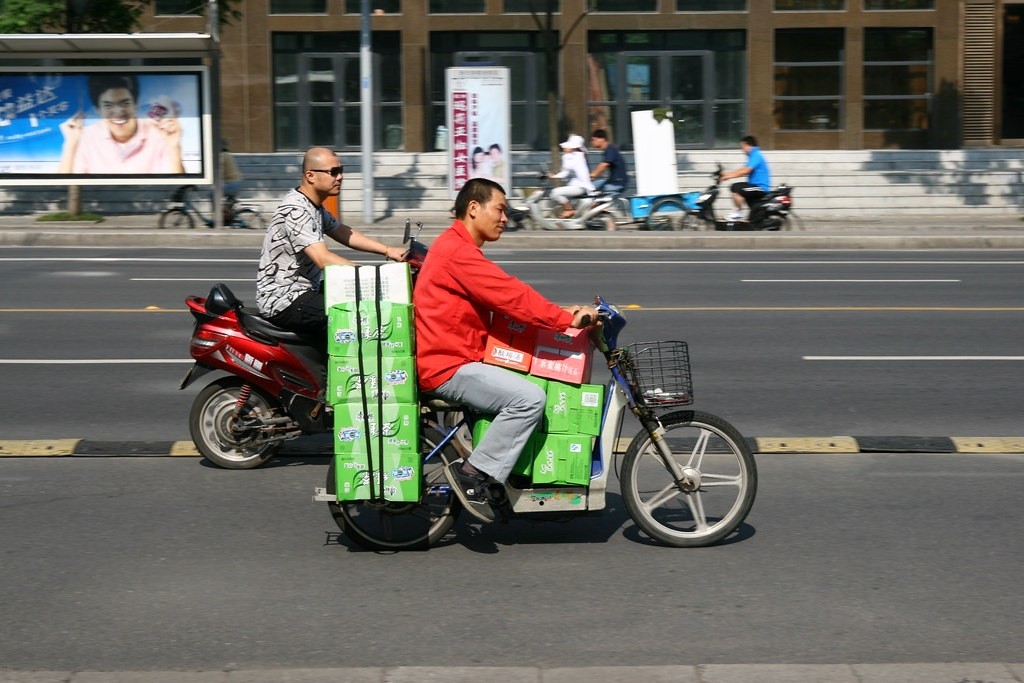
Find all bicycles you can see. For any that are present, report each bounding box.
[158,185,268,229]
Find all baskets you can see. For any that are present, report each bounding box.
[626,341,694,406]
[165,187,189,210]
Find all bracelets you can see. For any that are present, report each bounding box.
[386,246,389,261]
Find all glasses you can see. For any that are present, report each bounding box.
[312,165,344,177]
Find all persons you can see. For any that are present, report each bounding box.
[589,130,627,198]
[60,76,185,174]
[193,139,239,225]
[256,147,408,350]
[413,178,601,524]
[719,136,769,221]
[547,136,596,218]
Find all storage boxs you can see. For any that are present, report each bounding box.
[472,312,605,486]
[324,263,423,504]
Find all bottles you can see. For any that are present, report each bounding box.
[146,94,180,128]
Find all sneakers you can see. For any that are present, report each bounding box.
[444,462,494,523]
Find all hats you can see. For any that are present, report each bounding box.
[559,136,585,149]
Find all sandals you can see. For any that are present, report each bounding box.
[558,208,575,219]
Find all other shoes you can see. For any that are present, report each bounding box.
[724,212,745,219]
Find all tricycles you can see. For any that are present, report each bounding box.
[589,177,705,231]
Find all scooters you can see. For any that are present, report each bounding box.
[511,160,620,234]
[309,290,763,558]
[675,164,797,233]
[187,219,480,467]
